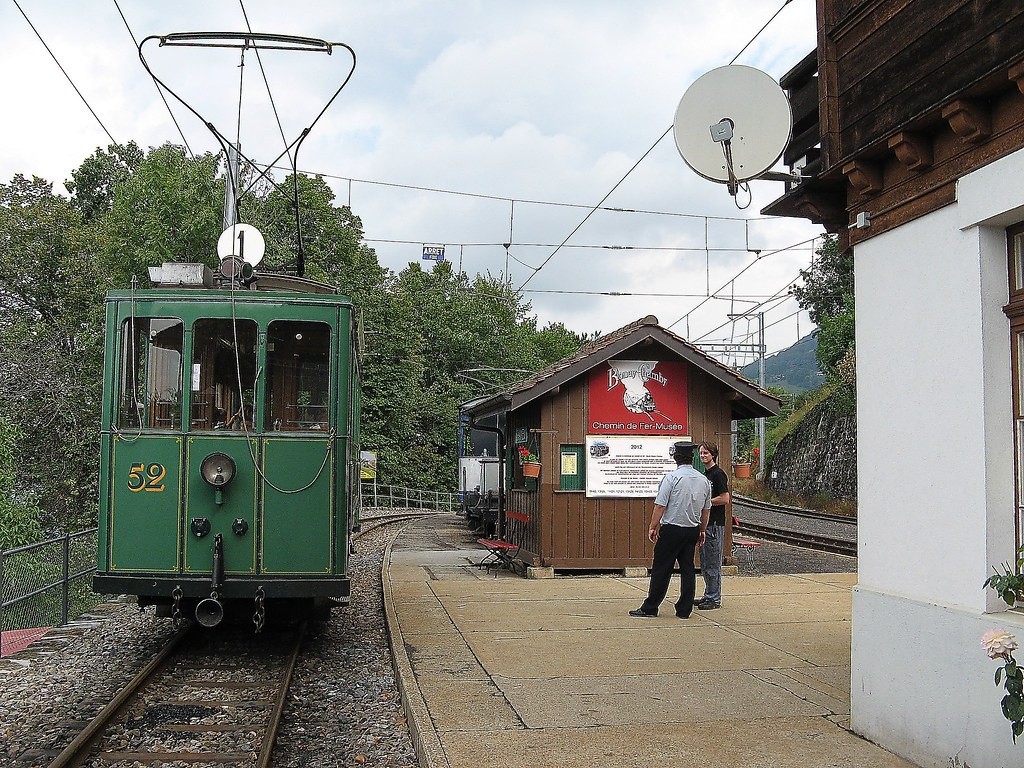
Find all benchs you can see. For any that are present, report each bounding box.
[731,514,761,569]
[476,510,529,579]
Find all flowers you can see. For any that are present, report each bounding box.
[518,444,539,463]
[731,448,760,466]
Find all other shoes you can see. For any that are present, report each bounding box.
[694,598,706,607]
[629,608,657,617]
[676,612,689,619]
[698,601,722,610]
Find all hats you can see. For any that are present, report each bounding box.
[675,442,695,452]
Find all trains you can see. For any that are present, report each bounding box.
[98,254,362,630]
[456,392,505,536]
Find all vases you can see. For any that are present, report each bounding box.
[732,464,749,478]
[523,461,541,478]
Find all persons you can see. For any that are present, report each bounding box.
[694,442,730,611]
[629,441,712,619]
[304,400,325,429]
